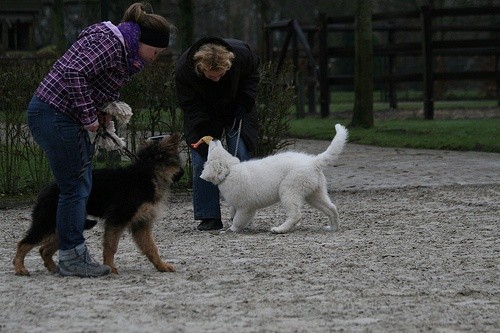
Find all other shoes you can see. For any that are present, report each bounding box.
[196,218,225,234]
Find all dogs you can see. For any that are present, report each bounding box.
[12,131,185,276]
[199,124,347,234]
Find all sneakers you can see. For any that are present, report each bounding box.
[57,253,112,277]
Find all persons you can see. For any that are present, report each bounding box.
[176,36,260,230]
[26,3,173,276]
